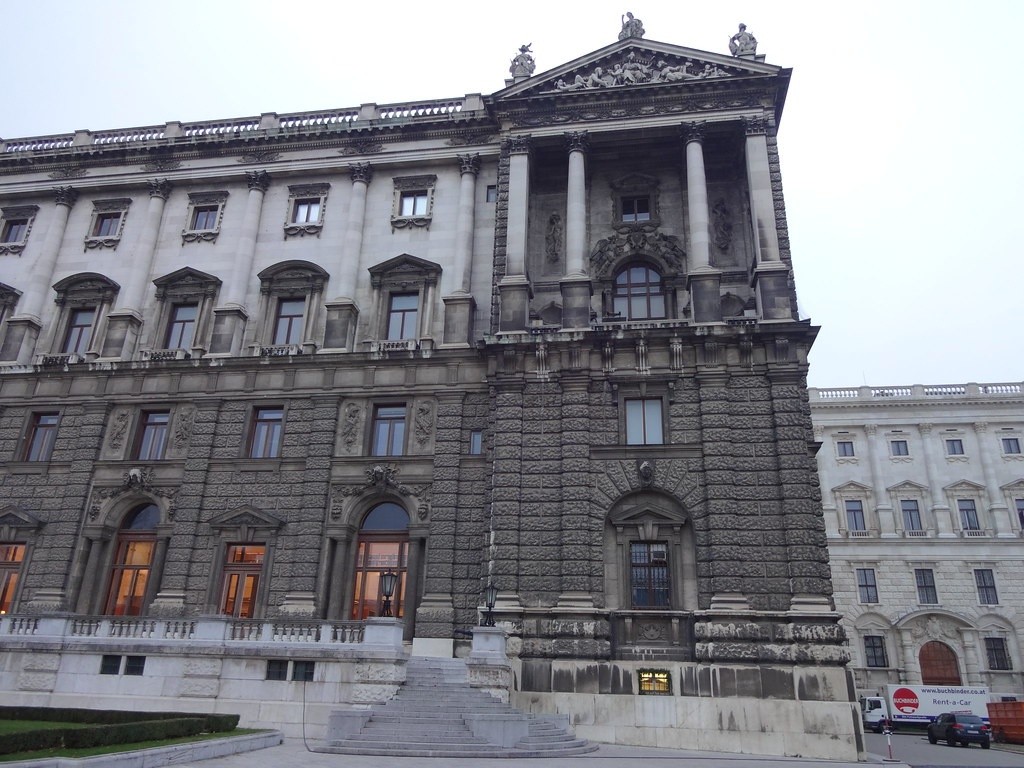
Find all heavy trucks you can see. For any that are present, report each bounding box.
[860,684,992,734]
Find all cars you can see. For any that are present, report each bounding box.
[927,713,990,749]
